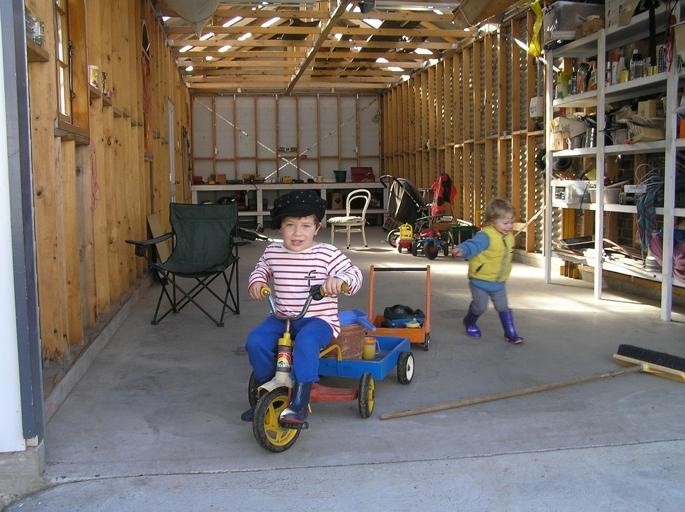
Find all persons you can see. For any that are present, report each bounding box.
[240,190,364,427]
[452,197,526,345]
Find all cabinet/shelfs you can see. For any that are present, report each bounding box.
[191,182,390,230]
[542,0,685,322]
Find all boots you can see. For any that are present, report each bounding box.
[499,309,525,345]
[279,381,312,424]
[463,301,482,337]
[241,407,255,422]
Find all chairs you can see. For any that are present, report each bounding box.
[125,201,250,328]
[327,189,372,249]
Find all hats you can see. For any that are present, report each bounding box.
[270,190,328,230]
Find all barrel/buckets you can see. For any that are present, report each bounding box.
[323,322,366,360]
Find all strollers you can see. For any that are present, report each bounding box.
[379,174,430,249]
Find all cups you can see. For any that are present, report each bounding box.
[363,337,377,360]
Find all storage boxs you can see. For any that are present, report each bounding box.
[540,1,604,50]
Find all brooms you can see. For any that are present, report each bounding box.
[379,343,685,417]
